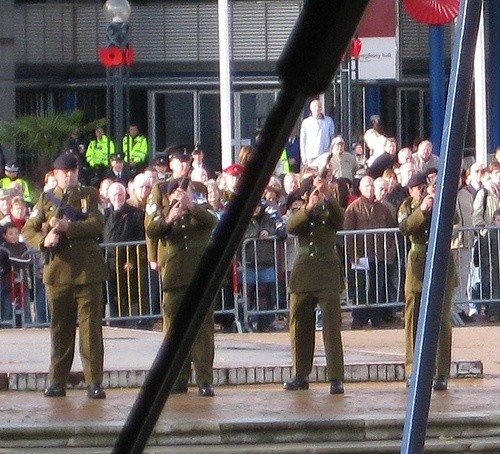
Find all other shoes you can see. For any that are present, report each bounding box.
[351,313,401,329]
[458,310,473,321]
[489,315,498,322]
[468,308,478,316]
[257,317,288,332]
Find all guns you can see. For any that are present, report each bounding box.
[419,175,438,203]
[308,152,335,202]
[160,156,196,248]
[42,181,73,265]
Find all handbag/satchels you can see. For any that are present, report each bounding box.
[474,240,480,268]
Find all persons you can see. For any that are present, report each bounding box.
[282,175,346,394]
[144,144,219,396]
[0,99,500,334]
[21,153,111,399]
[397,173,460,391]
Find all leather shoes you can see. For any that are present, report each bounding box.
[171,383,188,393]
[330,380,344,394]
[283,377,309,391]
[198,384,214,396]
[433,376,447,390]
[87,384,106,399]
[407,374,411,387]
[44,384,66,397]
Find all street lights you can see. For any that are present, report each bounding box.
[100,0,133,171]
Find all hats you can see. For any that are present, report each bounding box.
[408,174,426,188]
[224,163,245,176]
[110,152,126,161]
[53,154,77,169]
[0,188,13,200]
[300,177,320,197]
[5,162,20,172]
[164,143,195,157]
[285,188,304,208]
[152,152,167,164]
[193,146,204,154]
